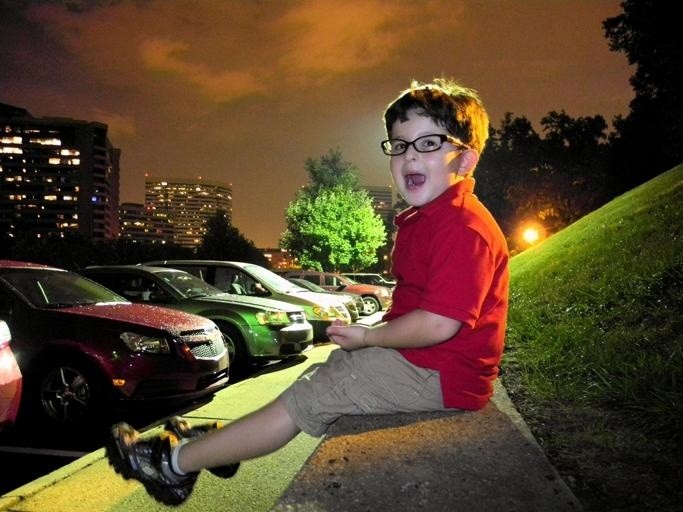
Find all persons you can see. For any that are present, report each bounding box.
[107,78,510,506]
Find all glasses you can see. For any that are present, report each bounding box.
[381,135,468,156]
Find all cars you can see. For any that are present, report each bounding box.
[287,277,364,315]
[79,263,313,364]
[0,258,229,431]
[310,292,359,323]
[0,318,22,425]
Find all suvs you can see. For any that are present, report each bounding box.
[341,273,396,289]
[281,270,392,315]
[141,260,352,341]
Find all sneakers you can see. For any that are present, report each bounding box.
[106,422,200,506]
[165,416,240,478]
